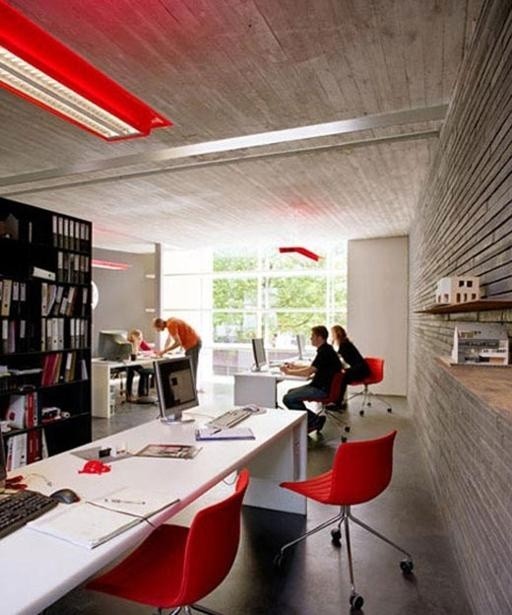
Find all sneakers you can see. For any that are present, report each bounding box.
[308,416,326,434]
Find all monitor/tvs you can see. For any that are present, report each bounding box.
[252,338,267,372]
[98,329,133,361]
[296,334,309,360]
[153,356,199,424]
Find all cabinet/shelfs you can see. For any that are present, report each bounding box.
[0,197,92,473]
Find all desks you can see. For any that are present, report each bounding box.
[234,358,314,409]
[92,350,186,420]
[0,404,307,615]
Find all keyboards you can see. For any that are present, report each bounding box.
[205,409,251,429]
[0,490,58,540]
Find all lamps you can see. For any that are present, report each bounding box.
[92,259,129,272]
[279,246,321,263]
[0,0,174,143]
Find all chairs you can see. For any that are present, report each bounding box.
[83,467,251,615]
[299,371,349,444]
[347,357,392,417]
[276,429,415,611]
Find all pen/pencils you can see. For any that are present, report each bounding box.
[105,498,146,506]
[210,428,222,435]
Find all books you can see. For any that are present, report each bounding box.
[27,485,180,550]
[1,213,90,474]
[195,426,256,441]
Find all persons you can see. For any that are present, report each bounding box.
[280,325,342,435]
[123,328,159,404]
[327,325,370,411]
[152,315,202,394]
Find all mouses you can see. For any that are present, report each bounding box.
[50,488,79,504]
[243,404,258,412]
